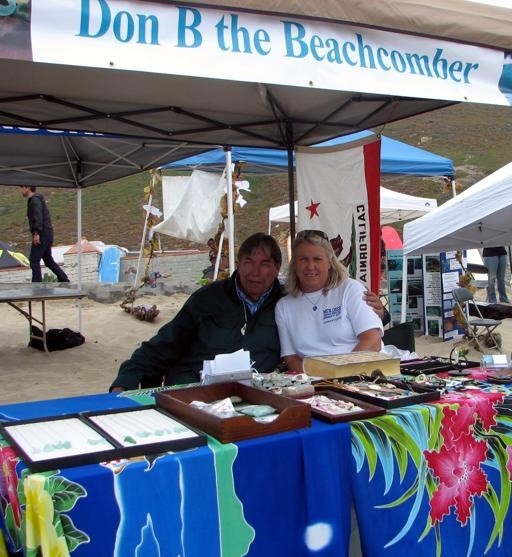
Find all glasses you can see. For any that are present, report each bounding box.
[315,230,329,241]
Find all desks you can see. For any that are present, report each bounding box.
[0,281,92,355]
[1,356,512,555]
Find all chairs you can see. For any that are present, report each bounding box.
[453,288,502,354]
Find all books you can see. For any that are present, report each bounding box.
[302,350,401,380]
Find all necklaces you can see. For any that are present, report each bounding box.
[298,287,329,313]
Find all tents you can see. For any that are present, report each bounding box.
[381,226,404,251]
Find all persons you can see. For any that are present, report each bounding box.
[110,233,392,393]
[20,184,70,283]
[483,246,511,305]
[274,228,386,376]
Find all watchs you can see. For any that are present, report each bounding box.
[32,231,39,235]
